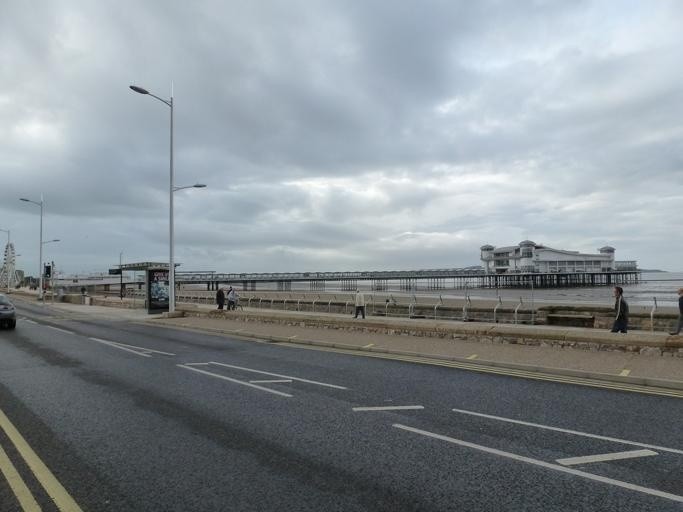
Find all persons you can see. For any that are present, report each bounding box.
[611,287,629,333]
[29,282,47,293]
[216,287,240,310]
[352,289,365,319]
[81,285,86,294]
[675,287,683,332]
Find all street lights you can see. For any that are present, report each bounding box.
[130,85,206,311]
[20,199,60,299]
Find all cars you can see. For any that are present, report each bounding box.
[0,294,17,329]
